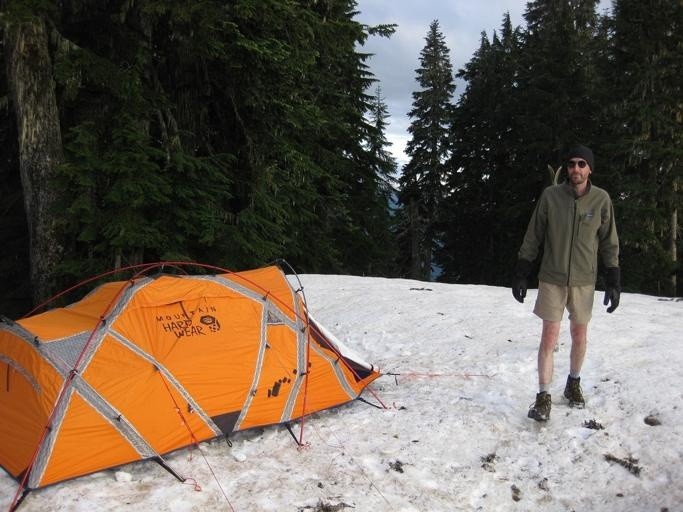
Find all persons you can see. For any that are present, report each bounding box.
[511,145,621,420]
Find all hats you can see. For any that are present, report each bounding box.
[568,144,593,171]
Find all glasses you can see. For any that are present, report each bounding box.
[568,161,586,168]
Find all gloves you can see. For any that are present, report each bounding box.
[603,268,620,313]
[511,260,536,302]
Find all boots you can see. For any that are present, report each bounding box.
[528,391,551,421]
[564,374,585,409]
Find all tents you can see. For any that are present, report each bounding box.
[0,257,387,493]
